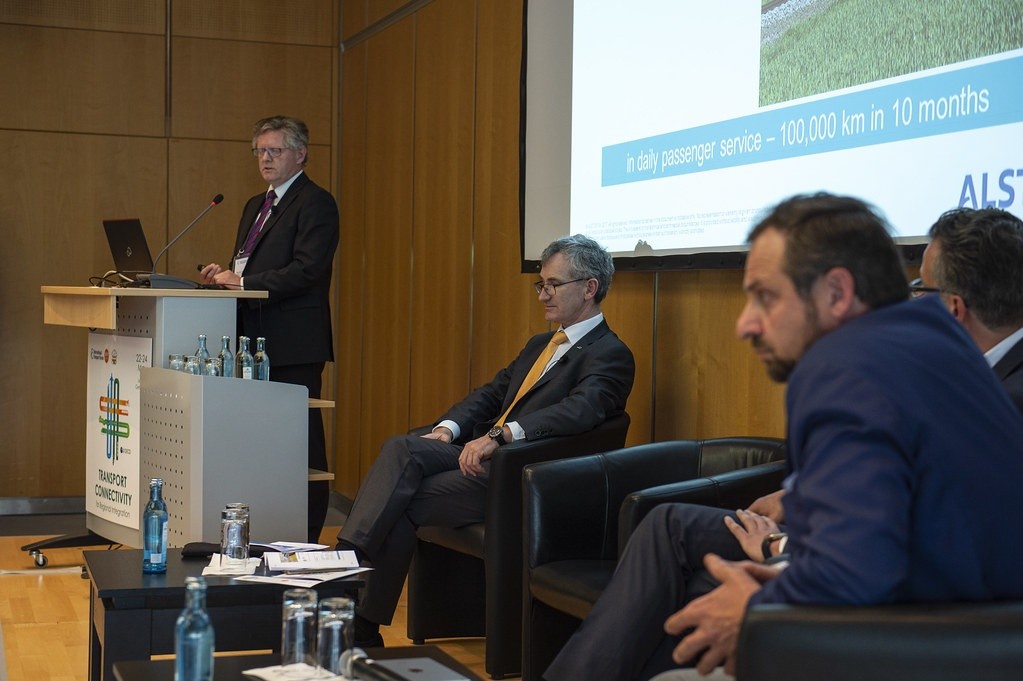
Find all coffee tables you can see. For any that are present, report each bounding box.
[81,546,369,681]
[111,644,489,681]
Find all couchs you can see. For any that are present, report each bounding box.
[404,412,630,676]
[733,601,1023,681]
[520,432,789,681]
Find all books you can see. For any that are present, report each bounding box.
[259,551,359,572]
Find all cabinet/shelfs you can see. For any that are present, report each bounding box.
[307,398,336,482]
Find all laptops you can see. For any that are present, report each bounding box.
[102,219,155,281]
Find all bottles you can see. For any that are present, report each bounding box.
[217,336,235,377]
[193,335,211,376]
[174,575,215,681]
[141,478,169,575]
[234,336,270,381]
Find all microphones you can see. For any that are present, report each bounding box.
[339,650,408,681]
[147,194,224,289]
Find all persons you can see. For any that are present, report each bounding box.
[201,114,340,544]
[334,233,635,648]
[540,189,1023,681]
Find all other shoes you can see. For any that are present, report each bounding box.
[355,633,385,647]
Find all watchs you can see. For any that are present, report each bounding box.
[761,532,787,560]
[488,425,507,446]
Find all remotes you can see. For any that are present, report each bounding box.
[197,264,210,274]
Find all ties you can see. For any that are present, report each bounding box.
[241,190,277,257]
[486,332,568,435]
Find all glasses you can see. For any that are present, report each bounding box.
[252,147,288,158]
[908,278,969,309]
[534,278,591,297]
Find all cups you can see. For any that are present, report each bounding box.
[219,503,252,573]
[168,353,221,377]
[281,587,357,681]
